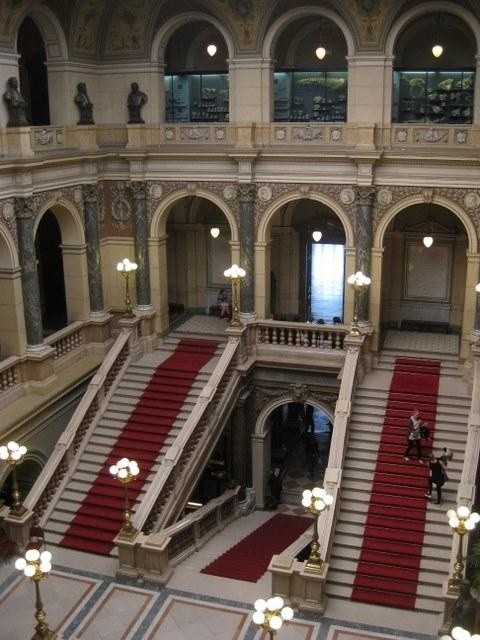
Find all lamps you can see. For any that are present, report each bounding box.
[206,41,216,57]
[431,45,444,58]
[315,44,325,60]
[312,228,323,242]
[422,235,434,248]
[210,227,220,238]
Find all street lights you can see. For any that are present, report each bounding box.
[0,440,28,514]
[109,456,142,532]
[253,595,295,639]
[441,625,480,640]
[346,271,372,330]
[117,254,140,319]
[301,485,334,567]
[444,508,480,595]
[15,548,53,638]
[223,262,248,326]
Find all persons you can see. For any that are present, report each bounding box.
[267,466,282,508]
[304,435,323,478]
[402,408,428,464]
[75,82,91,107]
[127,82,148,107]
[417,452,446,506]
[4,77,27,105]
[216,287,232,320]
[303,404,316,434]
